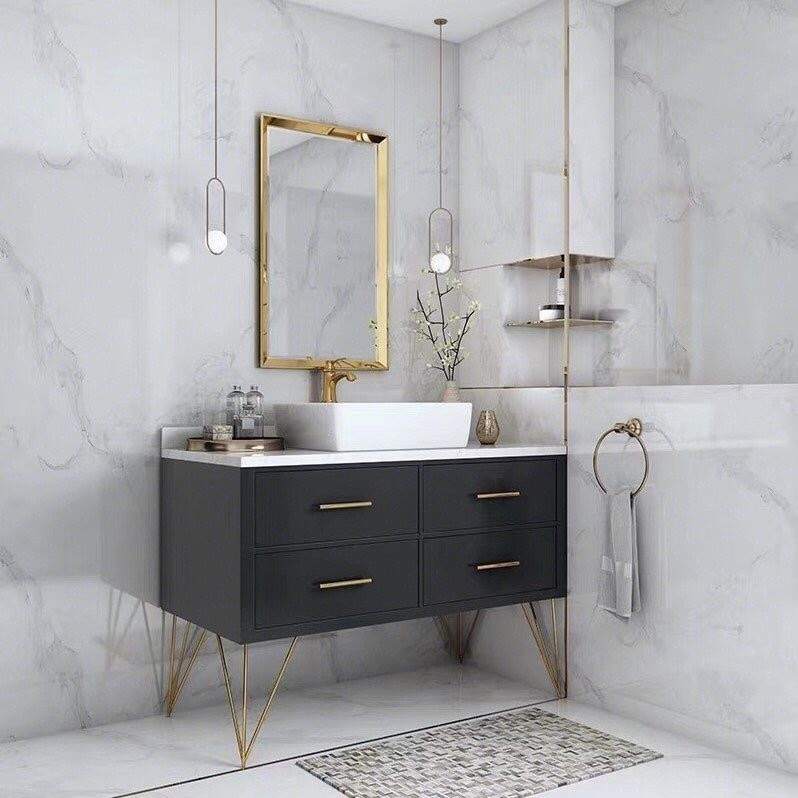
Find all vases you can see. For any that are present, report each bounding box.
[443,380,461,402]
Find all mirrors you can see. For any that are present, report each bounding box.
[260,113,389,369]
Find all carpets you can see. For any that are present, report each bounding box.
[296,707,663,798]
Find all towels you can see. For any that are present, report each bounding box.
[596,489,642,620]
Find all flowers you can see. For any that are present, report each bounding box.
[409,271,480,381]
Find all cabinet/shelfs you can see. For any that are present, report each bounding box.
[160,446,568,770]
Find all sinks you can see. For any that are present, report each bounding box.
[273,402,475,452]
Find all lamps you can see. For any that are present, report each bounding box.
[429,18,453,274]
[205,0,226,255]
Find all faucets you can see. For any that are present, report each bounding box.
[319,356,358,403]
[305,356,313,360]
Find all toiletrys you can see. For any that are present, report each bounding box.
[556,267,567,303]
[232,403,265,438]
[537,303,570,320]
